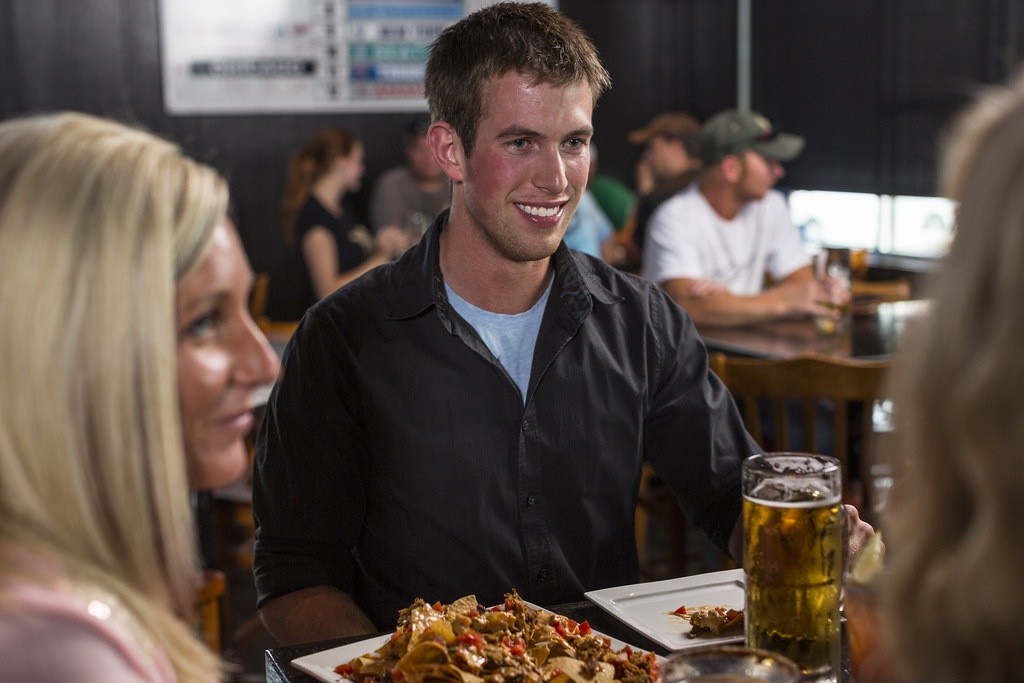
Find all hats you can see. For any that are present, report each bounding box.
[694,110,806,165]
[626,112,697,144]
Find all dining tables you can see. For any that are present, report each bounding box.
[263,548,912,682]
[685,307,910,522]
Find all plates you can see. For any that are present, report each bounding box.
[291,599,701,683]
[582,563,749,651]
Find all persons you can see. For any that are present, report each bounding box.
[370,128,456,260]
[0,112,281,683]
[578,144,638,275]
[866,68,1023,683]
[600,118,711,273]
[247,4,887,683]
[556,180,624,273]
[639,112,852,326]
[258,123,406,327]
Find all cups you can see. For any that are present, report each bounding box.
[656,643,800,682]
[744,451,863,682]
